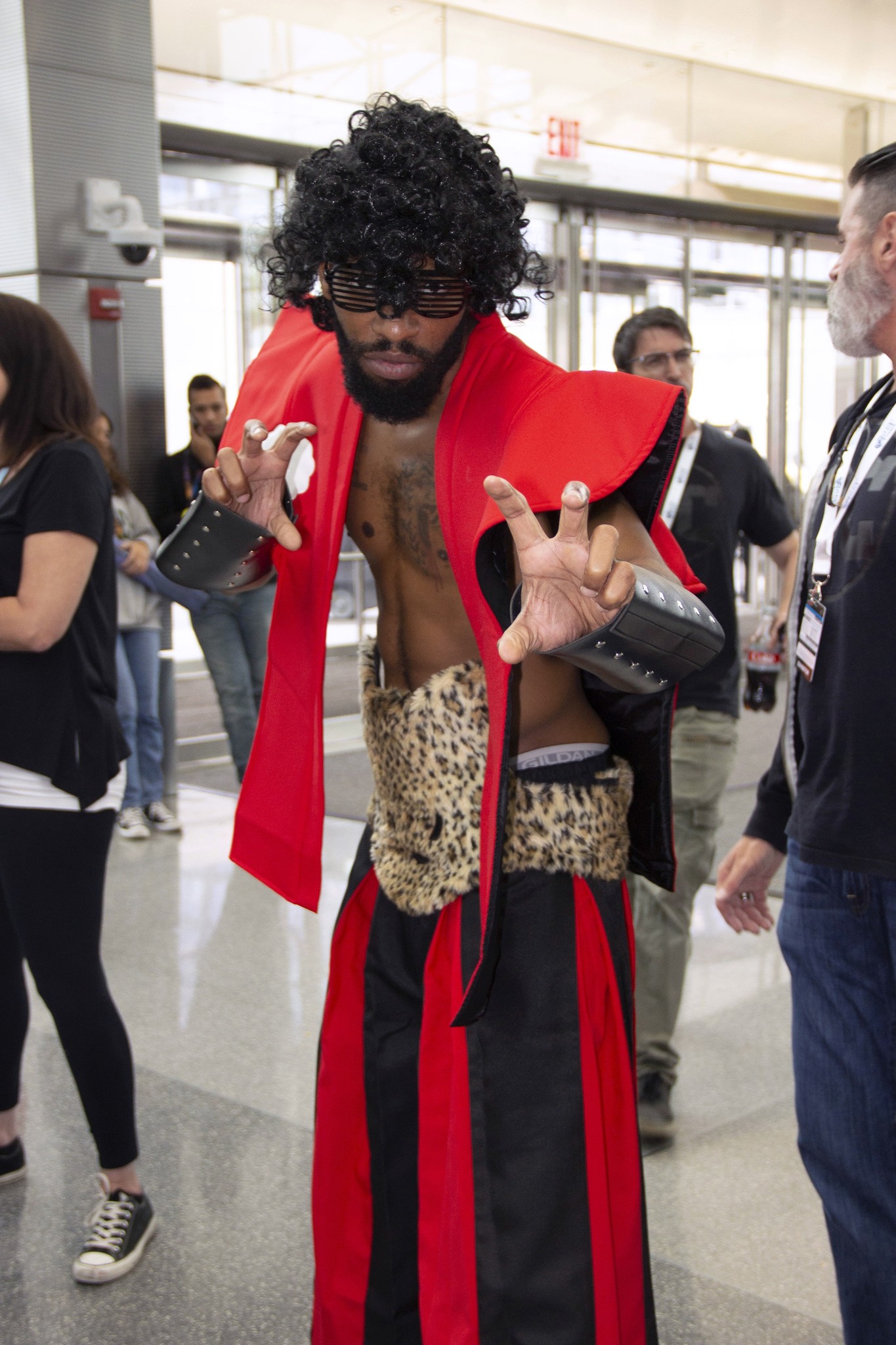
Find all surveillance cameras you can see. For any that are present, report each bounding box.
[110,220,164,265]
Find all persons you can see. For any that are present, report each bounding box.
[0,297,157,1282]
[154,90,726,1345]
[716,143,896,1345]
[92,375,279,837]
[613,306,804,1144]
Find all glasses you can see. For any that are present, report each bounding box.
[326,258,467,321]
[625,347,699,371]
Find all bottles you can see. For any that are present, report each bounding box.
[743,606,780,713]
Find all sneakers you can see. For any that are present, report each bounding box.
[1,1137,26,1183]
[72,1187,161,1285]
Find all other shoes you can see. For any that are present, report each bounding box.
[638,1069,681,1140]
[117,799,181,840]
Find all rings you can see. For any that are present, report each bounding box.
[740,892,754,902]
